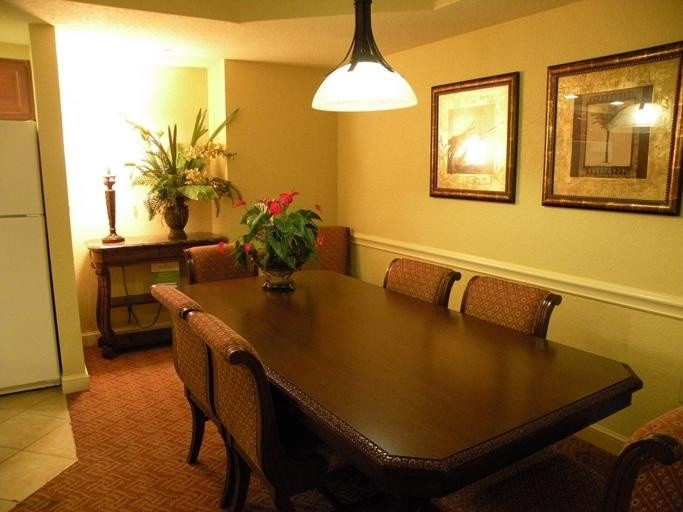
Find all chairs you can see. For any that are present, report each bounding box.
[182,243,246,283]
[146,283,234,512]
[459,275,561,342]
[384,258,462,310]
[599,405,683,511]
[183,311,345,511]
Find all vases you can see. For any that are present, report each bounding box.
[164,205,189,240]
[251,257,303,293]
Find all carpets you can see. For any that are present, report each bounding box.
[11,337,622,512]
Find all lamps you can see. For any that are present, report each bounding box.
[311,0,418,112]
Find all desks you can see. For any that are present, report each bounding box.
[177,267,643,512]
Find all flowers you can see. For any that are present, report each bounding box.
[123,108,246,227]
[218,191,325,276]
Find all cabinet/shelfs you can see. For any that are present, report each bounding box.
[84,231,228,361]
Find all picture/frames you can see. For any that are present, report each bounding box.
[429,72,520,204]
[1,61,34,121]
[541,40,683,217]
